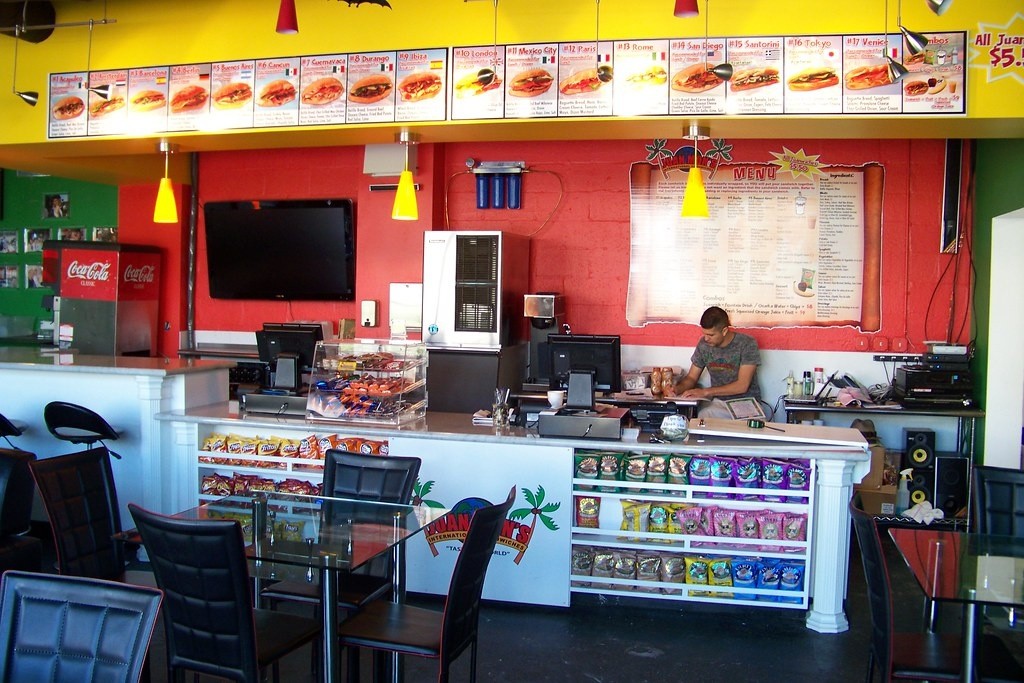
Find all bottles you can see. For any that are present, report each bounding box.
[787,366,824,399]
[649,367,673,394]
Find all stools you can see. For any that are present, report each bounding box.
[0,400,124,450]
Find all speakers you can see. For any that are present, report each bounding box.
[898,428,970,518]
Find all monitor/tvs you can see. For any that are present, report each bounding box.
[204,198,355,303]
[547,333,622,413]
[263,323,326,393]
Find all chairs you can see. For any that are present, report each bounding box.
[966,466,1024,629]
[0,569,164,683]
[127,449,517,683]
[0,449,43,584]
[849,492,1024,683]
[28,446,159,683]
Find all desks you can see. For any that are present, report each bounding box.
[887,527,1024,683]
[110,490,453,683]
[783,396,985,526]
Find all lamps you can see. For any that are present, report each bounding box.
[680,125,711,218]
[86,19,113,102]
[12,25,39,108]
[153,143,179,224]
[674,0,699,18]
[276,0,299,35]
[391,131,420,221]
[883,0,952,84]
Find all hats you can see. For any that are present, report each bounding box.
[850,418,881,439]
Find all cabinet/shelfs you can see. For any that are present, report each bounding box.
[196,422,390,567]
[304,339,430,422]
[566,447,815,607]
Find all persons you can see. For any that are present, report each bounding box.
[50,195,62,217]
[9,238,16,250]
[9,276,17,288]
[28,237,43,250]
[64,229,83,240]
[674,306,761,419]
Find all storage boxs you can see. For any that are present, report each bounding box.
[859,485,897,516]
[851,439,884,491]
